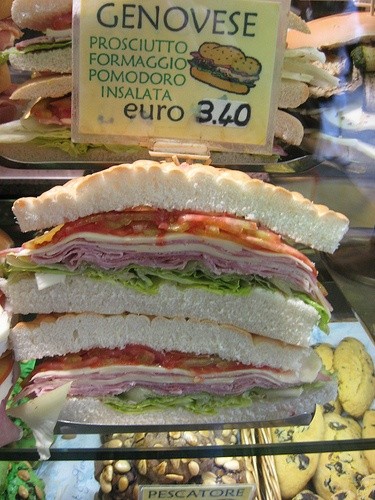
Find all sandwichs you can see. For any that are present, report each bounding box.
[0,159,352,350]
[7,315,340,426]
[2,76,311,168]
[1,0,336,108]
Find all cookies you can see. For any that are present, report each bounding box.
[1,337,375,500]
